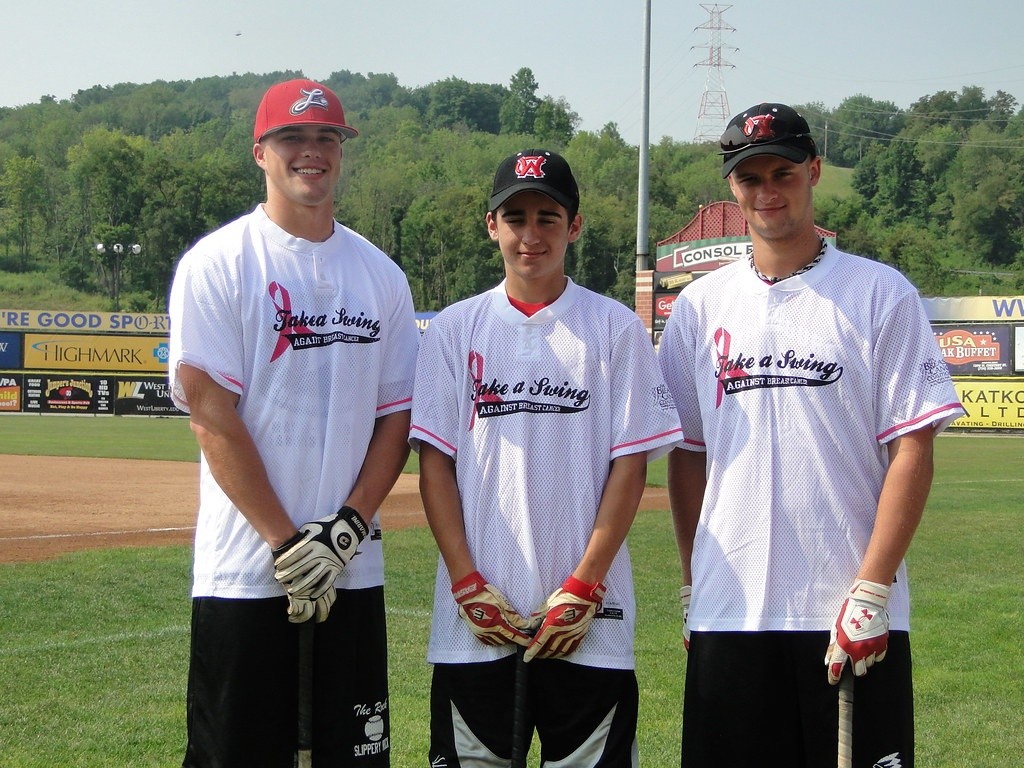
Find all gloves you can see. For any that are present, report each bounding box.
[452,571,534,648]
[271,534,338,625]
[823,579,891,686]
[677,585,693,651]
[523,573,607,664]
[274,503,370,603]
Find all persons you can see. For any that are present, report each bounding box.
[407,150,684,768]
[169,79,422,768]
[656,102,966,768]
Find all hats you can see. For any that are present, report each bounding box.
[716,101,819,179]
[254,78,359,143]
[489,148,579,216]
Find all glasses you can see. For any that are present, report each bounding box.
[718,123,816,156]
